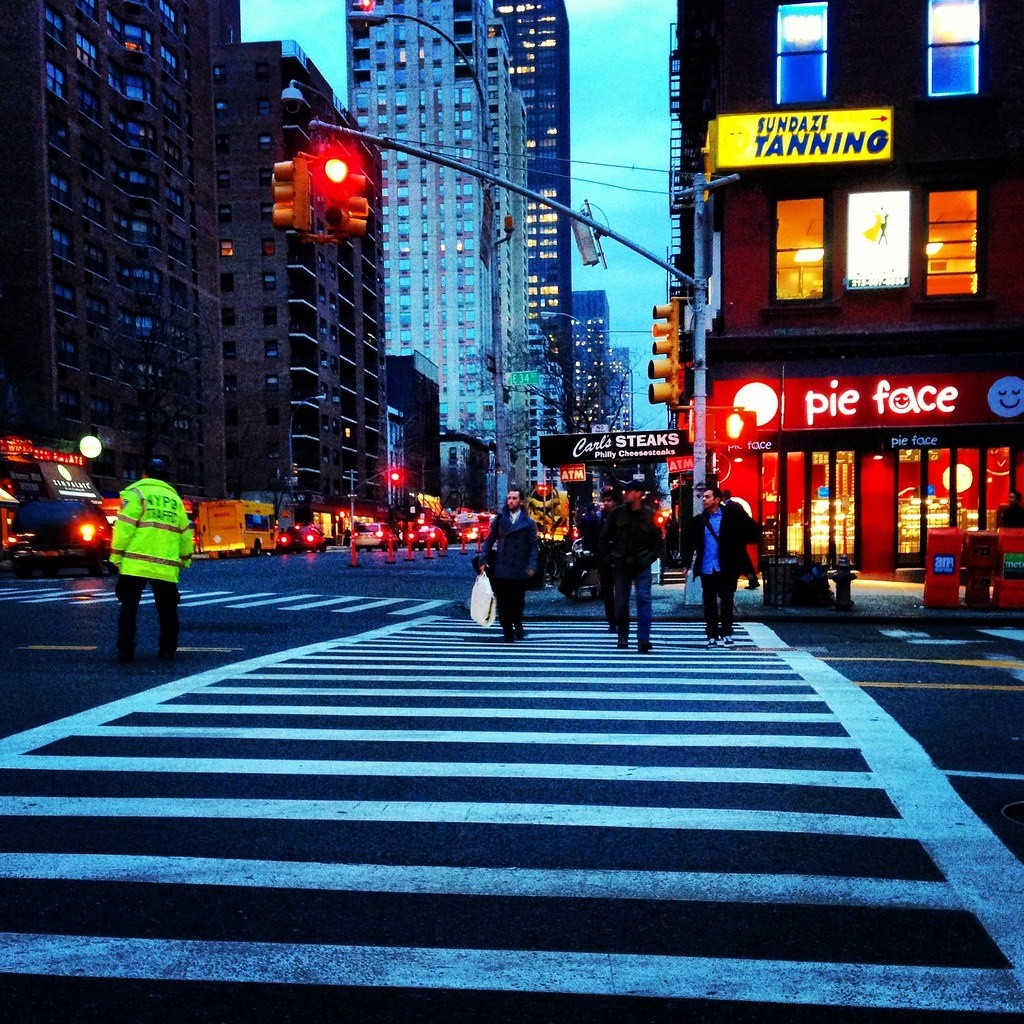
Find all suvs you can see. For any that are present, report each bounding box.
[0,495,118,579]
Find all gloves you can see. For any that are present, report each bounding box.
[106,562,118,575]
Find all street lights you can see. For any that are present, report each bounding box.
[349,11,509,511]
[288,395,326,527]
[540,312,603,518]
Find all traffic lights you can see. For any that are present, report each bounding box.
[349,174,369,237]
[325,159,349,231]
[648,300,679,408]
[272,160,312,232]
[391,472,401,481]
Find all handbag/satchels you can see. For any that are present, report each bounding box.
[472,550,495,577]
[470,570,497,629]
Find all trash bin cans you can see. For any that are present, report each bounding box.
[760,555,799,606]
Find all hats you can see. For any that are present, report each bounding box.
[145,458,168,475]
[620,479,648,491]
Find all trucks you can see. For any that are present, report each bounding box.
[453,512,496,544]
[200,500,278,558]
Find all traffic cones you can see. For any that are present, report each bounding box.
[827,553,858,611]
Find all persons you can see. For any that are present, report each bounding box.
[478,488,540,643]
[680,486,743,648]
[597,479,662,652]
[1002,490,1024,528]
[107,459,194,663]
[581,489,622,632]
[721,489,760,589]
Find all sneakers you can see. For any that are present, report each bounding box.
[707,637,717,647]
[721,635,735,647]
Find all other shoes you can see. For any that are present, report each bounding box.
[744,579,760,589]
[504,629,514,643]
[608,624,616,632]
[618,640,628,647]
[638,641,652,654]
[515,624,524,644]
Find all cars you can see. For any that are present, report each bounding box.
[354,522,399,553]
[410,527,447,551]
[276,523,326,554]
